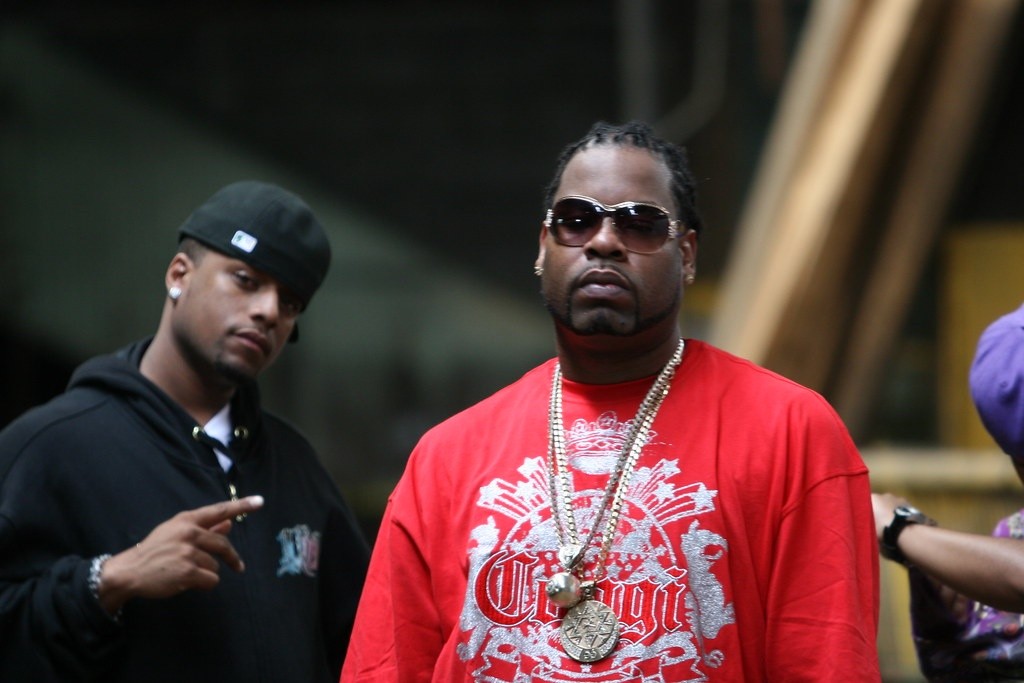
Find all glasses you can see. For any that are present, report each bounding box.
[543,194,685,256]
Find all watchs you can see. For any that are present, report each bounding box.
[883,505,937,568]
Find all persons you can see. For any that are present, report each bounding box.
[0,181,372,683]
[871,305,1024,683]
[340,122,880,683]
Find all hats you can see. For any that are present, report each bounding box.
[178,180,332,344]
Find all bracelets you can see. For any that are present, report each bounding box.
[88,554,112,600]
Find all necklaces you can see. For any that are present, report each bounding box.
[545,337,684,663]
